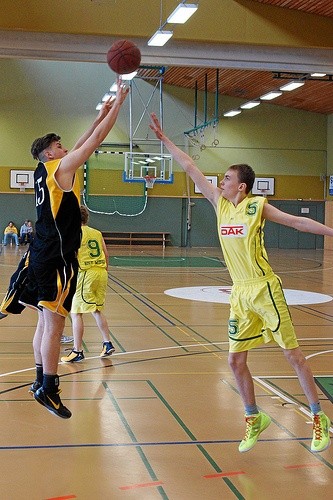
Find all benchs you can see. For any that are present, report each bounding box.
[101,230,171,248]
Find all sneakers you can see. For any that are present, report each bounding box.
[33,385,73,420]
[311,411,331,452]
[238,412,271,454]
[60,335,74,343]
[99,341,116,358]
[61,349,84,362]
[28,380,62,398]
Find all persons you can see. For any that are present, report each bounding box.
[0,74,130,419]
[149,112,333,455]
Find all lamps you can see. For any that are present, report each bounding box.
[148,0,200,46]
[223,72,326,117]
[96,71,137,110]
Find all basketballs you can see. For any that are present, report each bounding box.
[106,39,143,75]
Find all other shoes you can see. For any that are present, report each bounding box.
[4,243,7,246]
[16,243,20,246]
